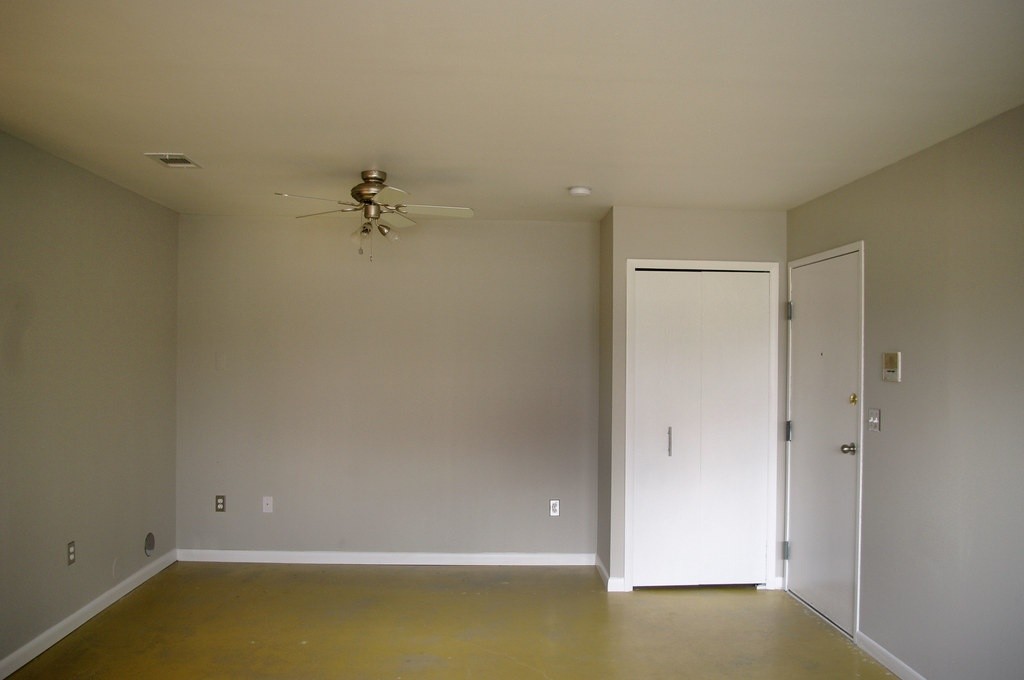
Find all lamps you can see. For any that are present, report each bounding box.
[353,203,403,263]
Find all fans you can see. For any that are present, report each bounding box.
[275,170,474,230]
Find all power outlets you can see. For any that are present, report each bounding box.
[262,496,273,514]
[216,494,226,513]
[549,499,560,518]
